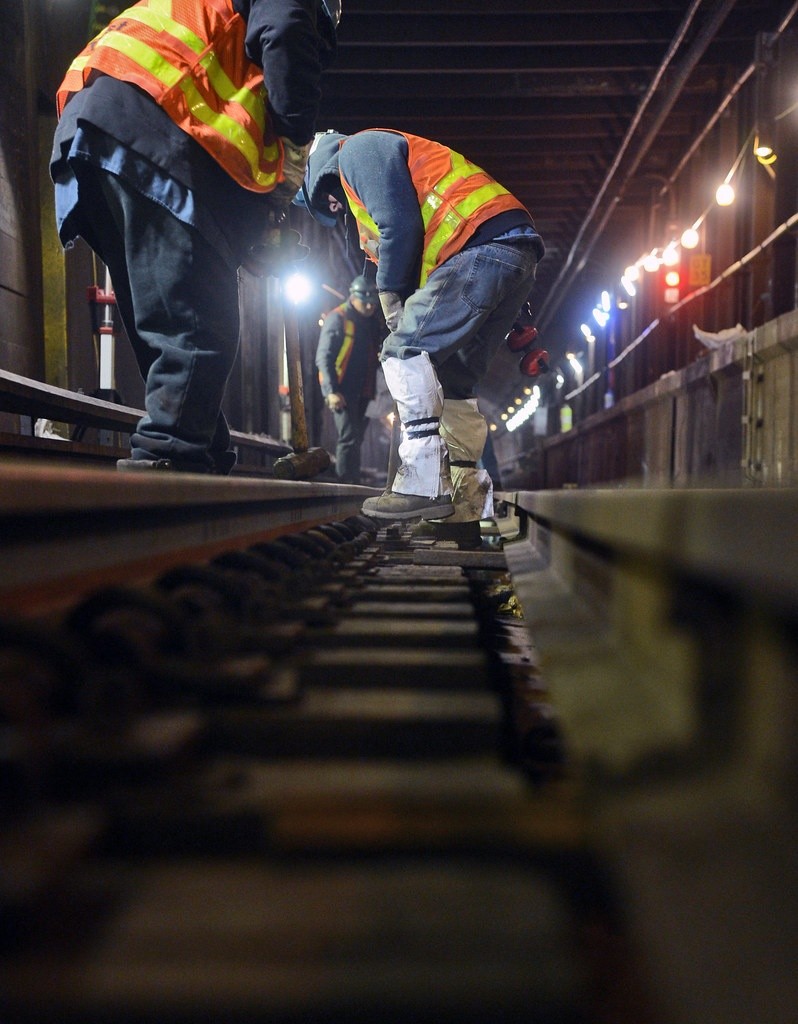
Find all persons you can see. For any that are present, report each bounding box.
[298,127,544,524]
[315,275,390,486]
[48,0,342,477]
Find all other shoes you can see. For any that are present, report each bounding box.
[116,459,171,472]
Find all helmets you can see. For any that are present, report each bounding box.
[352,274,379,303]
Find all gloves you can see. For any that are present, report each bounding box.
[378,292,406,333]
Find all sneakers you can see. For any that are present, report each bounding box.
[362,491,454,521]
[408,518,482,547]
